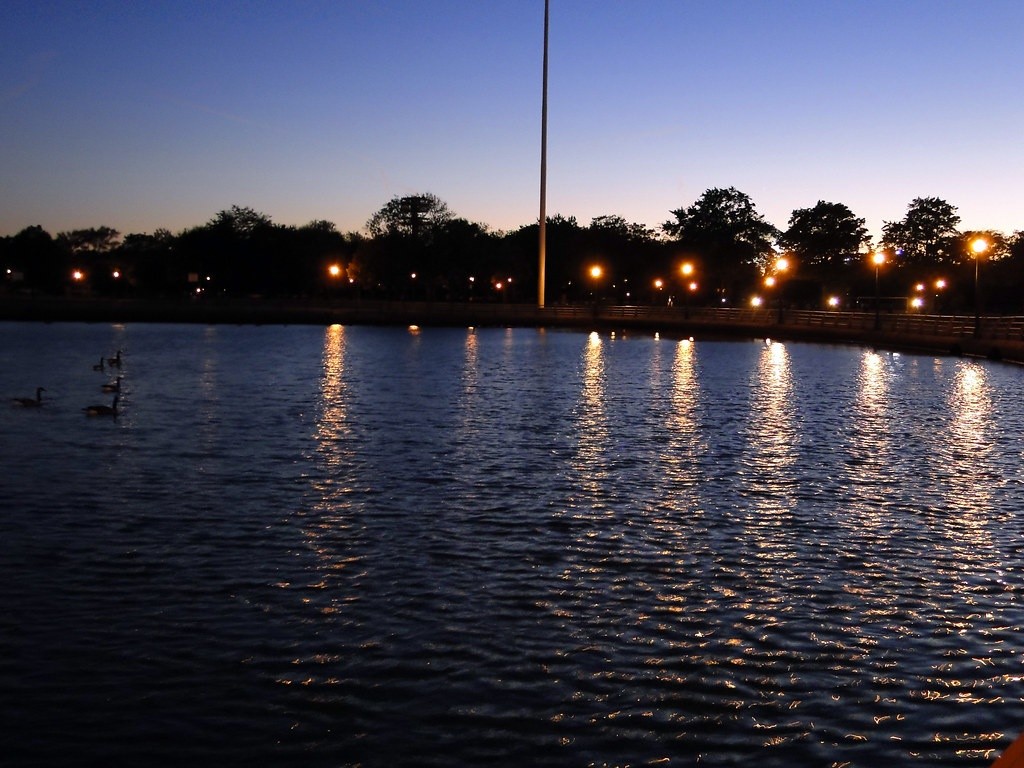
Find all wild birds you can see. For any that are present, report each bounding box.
[7,346,127,420]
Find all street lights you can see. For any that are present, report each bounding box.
[970,235,991,324]
[870,250,887,330]
